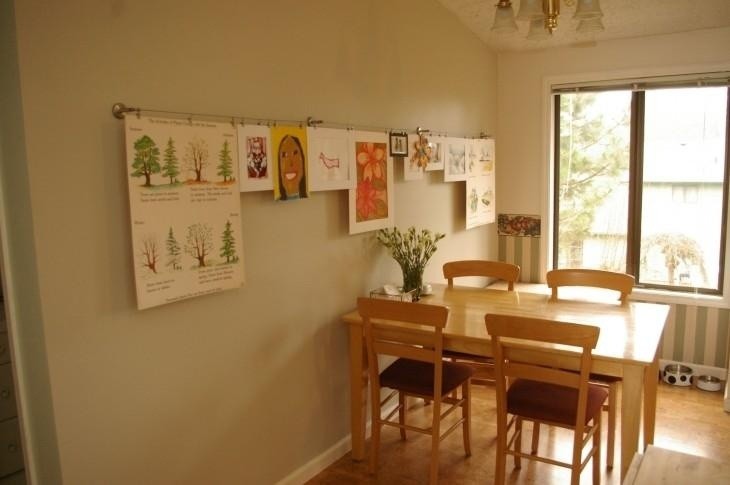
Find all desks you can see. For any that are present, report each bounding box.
[619,444,730,485]
[485,278,623,303]
[337,278,671,485]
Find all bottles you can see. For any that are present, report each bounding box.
[419,283,433,298]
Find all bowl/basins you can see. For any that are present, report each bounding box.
[662,362,694,388]
[694,371,722,392]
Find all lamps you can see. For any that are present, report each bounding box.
[490,0,605,40]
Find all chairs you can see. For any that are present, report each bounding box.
[543,269,636,469]
[355,297,474,485]
[485,312,608,485]
[442,258,521,407]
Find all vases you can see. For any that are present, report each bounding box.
[402,270,425,302]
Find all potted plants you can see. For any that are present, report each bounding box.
[376,224,447,298]
